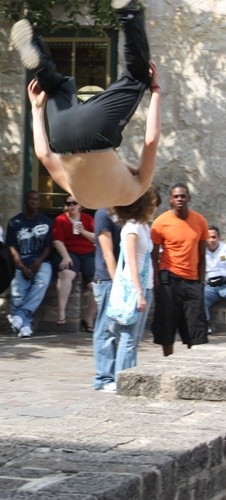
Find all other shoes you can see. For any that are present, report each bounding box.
[10,19,39,69]
[208,324,215,332]
[111,1,136,8]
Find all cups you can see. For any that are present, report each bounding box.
[73,221,82,234]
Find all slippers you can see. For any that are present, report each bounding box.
[81,319,94,333]
[57,319,67,325]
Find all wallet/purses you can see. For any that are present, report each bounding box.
[209,276,223,286]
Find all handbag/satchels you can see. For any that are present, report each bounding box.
[107,270,148,326]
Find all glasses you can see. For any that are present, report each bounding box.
[65,202,78,205]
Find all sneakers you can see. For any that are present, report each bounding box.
[7,310,23,333]
[95,382,116,392]
[18,326,32,337]
